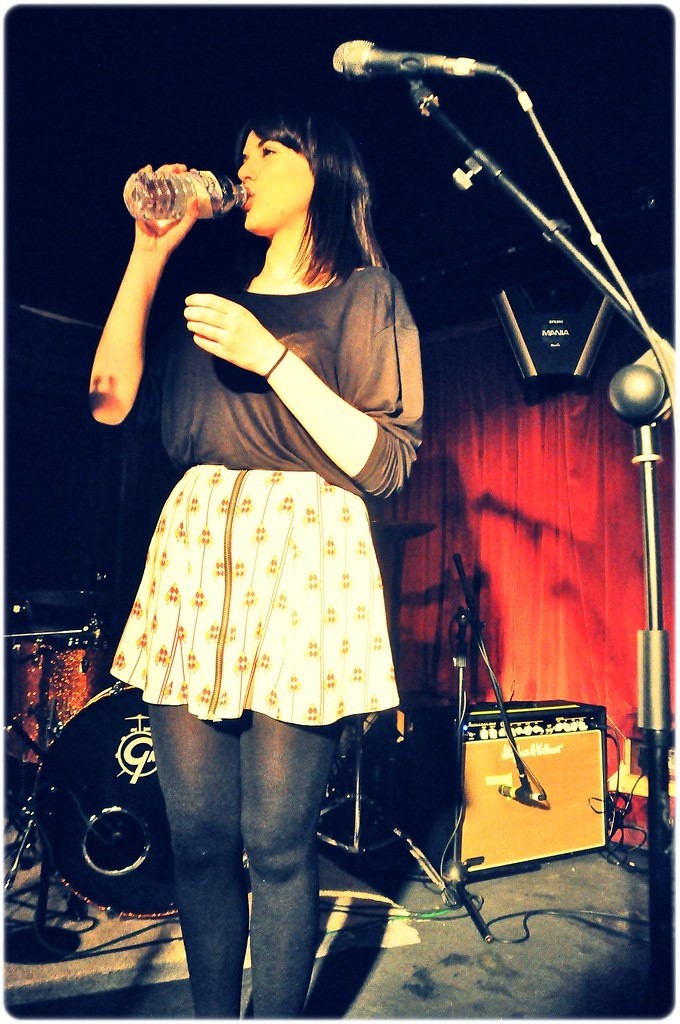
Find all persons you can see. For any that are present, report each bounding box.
[88,104,423,1020]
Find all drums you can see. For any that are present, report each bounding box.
[5,610,120,799]
[19,679,181,921]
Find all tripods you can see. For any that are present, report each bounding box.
[412,553,541,944]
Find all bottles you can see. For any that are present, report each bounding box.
[123,170,246,221]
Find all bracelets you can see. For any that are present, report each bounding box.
[265,349,287,380]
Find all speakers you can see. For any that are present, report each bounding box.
[416,699,608,881]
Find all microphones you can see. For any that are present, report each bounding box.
[332,40,498,80]
[498,785,546,801]
[107,832,122,849]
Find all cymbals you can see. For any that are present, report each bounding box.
[370,518,439,546]
[11,585,133,613]
